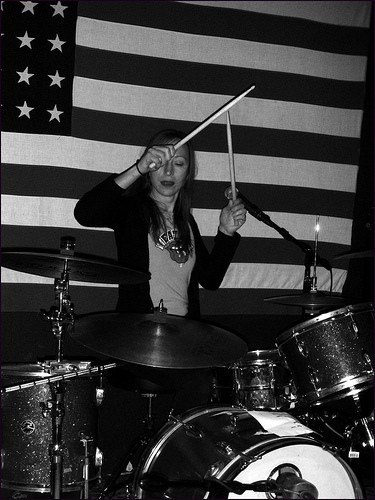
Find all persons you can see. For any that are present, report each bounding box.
[74,128,247,320]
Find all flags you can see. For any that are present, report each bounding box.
[0,0,372,314]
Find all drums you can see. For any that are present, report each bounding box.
[0,363,103,494]
[132,405,367,500]
[275,303,375,405]
[231,349,293,407]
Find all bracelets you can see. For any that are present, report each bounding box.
[136,159,143,176]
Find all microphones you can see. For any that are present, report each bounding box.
[276,472,319,499]
[224,186,264,222]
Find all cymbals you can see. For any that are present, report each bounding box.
[0,252,151,283]
[67,312,248,369]
[264,292,349,310]
[336,250,372,260]
